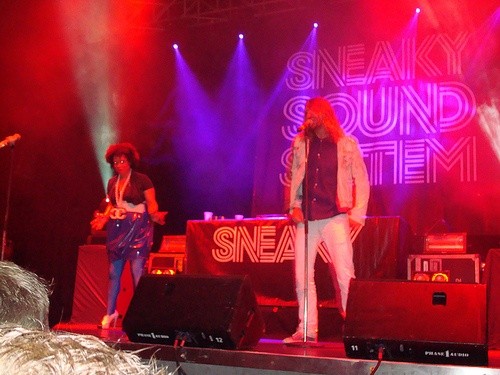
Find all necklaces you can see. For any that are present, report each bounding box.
[115,174,130,207]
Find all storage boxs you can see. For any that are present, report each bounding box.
[408,254,480,283]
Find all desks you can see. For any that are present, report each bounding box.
[187,217,400,306]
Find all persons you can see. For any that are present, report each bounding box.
[0,260,181,375]
[283,97,370,344]
[87,143,168,328]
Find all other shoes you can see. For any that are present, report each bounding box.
[284,332,318,342]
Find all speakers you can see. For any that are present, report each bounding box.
[341,278,489,366]
[122,274,264,350]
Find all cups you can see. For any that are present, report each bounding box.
[204,212,213,222]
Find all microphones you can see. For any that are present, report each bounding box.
[0,133,20,148]
[297,119,312,131]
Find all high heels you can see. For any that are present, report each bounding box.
[102,311,118,328]
